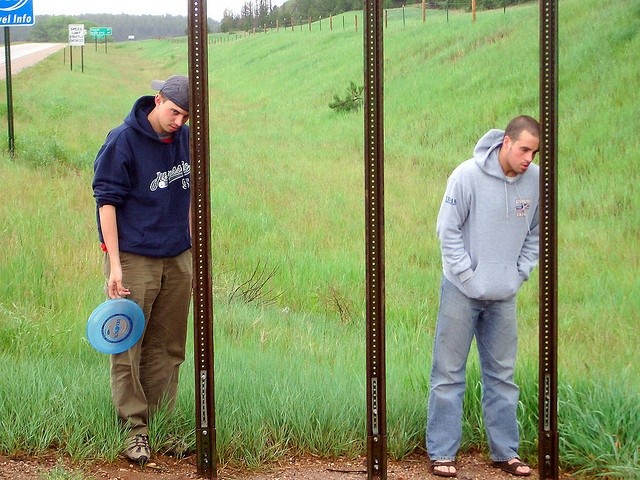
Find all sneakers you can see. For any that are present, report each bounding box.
[161,433,190,458]
[124,434,150,463]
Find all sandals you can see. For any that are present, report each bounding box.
[492,457,532,476]
[431,460,457,477]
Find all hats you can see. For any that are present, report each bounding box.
[151,75,188,112]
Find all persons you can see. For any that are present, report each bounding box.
[424,113,540,478]
[91,73,191,464]
